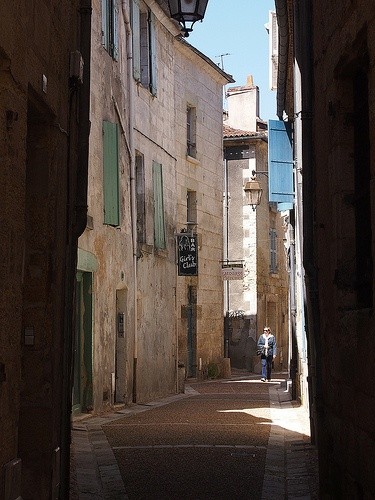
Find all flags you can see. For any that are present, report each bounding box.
[177,233,198,277]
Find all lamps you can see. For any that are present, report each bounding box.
[244,177,263,211]
[168,0,208,37]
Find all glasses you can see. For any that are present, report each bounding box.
[263,330,268,331]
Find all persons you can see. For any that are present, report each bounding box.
[256,326,277,381]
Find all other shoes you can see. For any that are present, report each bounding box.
[261,378,266,382]
[267,378,272,381]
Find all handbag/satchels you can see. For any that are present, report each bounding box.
[256,347,263,354]
